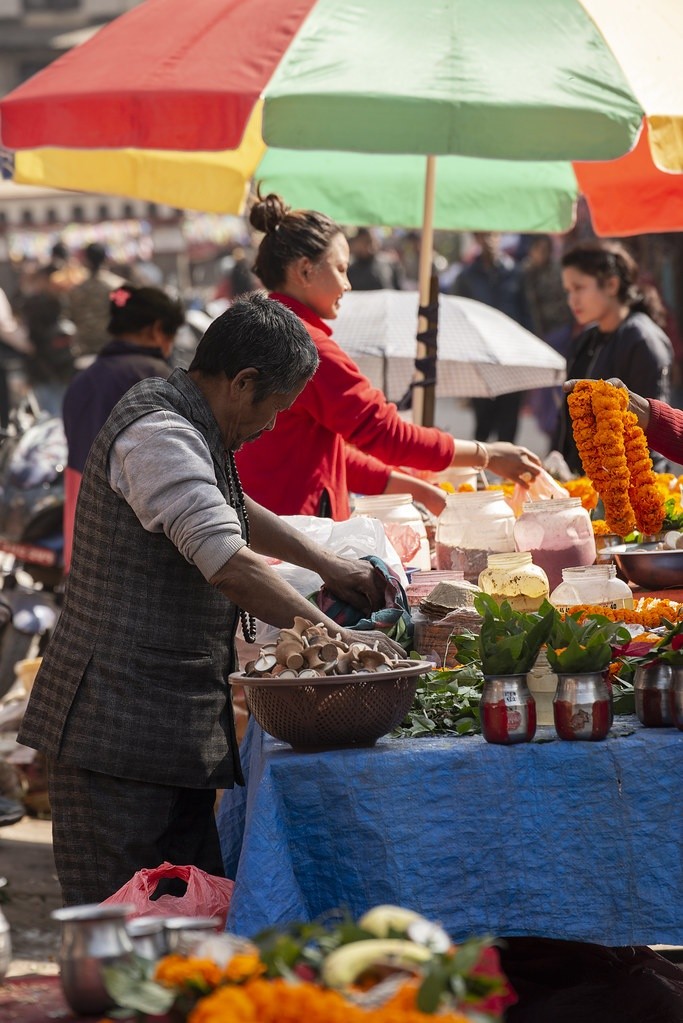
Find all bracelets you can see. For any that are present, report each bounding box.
[472,440,489,469]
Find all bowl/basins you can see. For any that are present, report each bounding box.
[598,542,683,590]
[593,534,631,584]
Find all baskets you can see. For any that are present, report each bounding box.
[227,659,431,749]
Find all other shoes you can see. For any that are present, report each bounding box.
[0,763,23,824]
[24,771,51,820]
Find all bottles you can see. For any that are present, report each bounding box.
[124,916,165,960]
[478,552,550,613]
[48,902,136,1016]
[551,669,612,742]
[0,876,14,980]
[479,672,538,744]
[434,489,521,587]
[404,571,465,620]
[163,916,221,959]
[551,564,632,608]
[349,493,431,571]
[514,498,598,598]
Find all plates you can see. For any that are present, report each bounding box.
[599,598,683,631]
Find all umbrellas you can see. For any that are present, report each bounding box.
[329,288,568,408]
[0,0,683,428]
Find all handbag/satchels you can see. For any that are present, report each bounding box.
[303,555,414,656]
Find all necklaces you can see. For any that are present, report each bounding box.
[224,449,257,644]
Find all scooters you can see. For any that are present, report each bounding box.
[0,416,69,699]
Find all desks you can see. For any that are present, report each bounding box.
[215,713,683,946]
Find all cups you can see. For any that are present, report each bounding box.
[633,666,683,732]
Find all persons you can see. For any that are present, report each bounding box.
[235,192,541,521]
[16,290,387,909]
[543,240,674,481]
[63,285,186,579]
[0,227,579,456]
[562,377,683,469]
